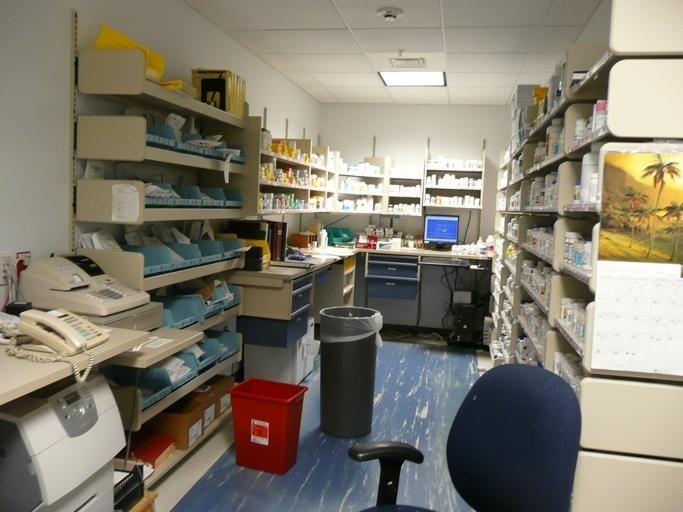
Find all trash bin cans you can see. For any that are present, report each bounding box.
[225,377,308,474]
[319,306,378,438]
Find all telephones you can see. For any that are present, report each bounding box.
[17,309,109,357]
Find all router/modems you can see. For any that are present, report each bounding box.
[452,291,472,304]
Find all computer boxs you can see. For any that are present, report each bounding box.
[454,292,481,347]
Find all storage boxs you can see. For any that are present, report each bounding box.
[151,374,234,450]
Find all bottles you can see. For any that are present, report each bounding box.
[476,235,495,257]
[311,229,328,249]
[426,158,482,207]
[387,204,421,214]
[506,100,608,392]
[259,126,323,210]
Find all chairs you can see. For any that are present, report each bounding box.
[346,362,581,511]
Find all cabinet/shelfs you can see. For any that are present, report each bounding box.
[260,106,496,216]
[485,0,683,512]
[77,48,263,434]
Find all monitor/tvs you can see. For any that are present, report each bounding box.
[423,214,459,251]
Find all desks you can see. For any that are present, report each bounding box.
[227,244,494,386]
[2,308,205,511]
